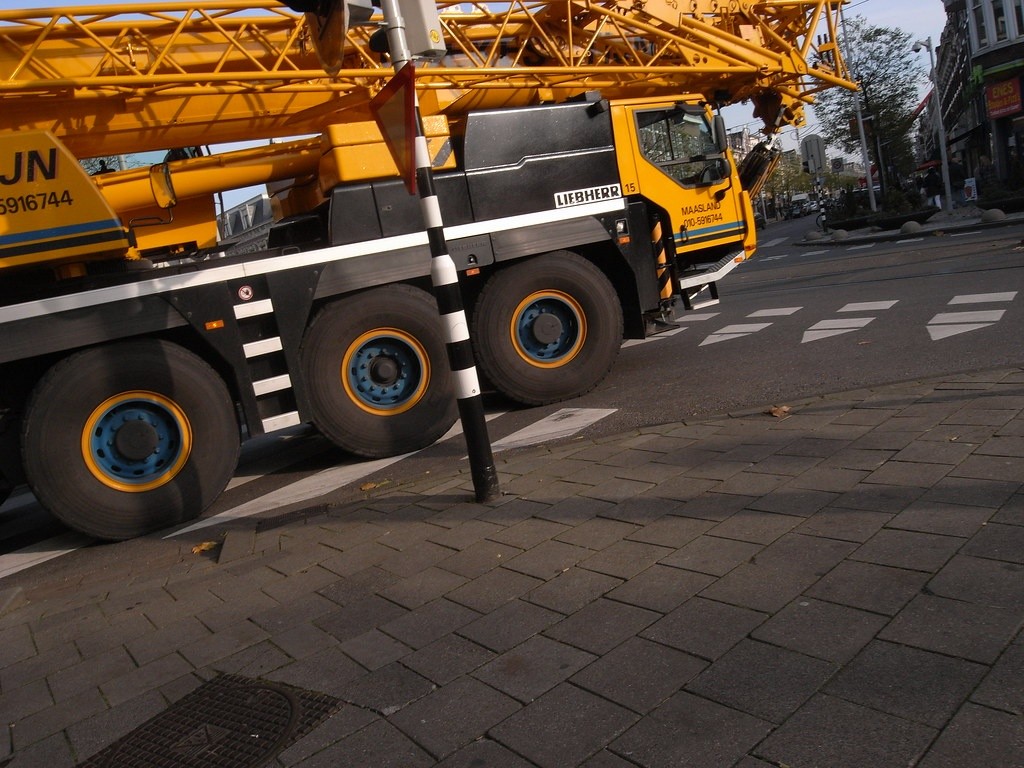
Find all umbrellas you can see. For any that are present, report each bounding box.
[917,160,942,172]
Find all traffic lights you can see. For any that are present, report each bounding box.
[802,160,817,175]
[366,0,447,63]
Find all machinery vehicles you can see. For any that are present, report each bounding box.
[1,1,863,546]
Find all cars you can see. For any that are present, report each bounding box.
[784,194,835,219]
[753,212,766,230]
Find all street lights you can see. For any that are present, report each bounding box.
[911,36,953,211]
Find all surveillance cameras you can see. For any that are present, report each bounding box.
[912,44,922,53]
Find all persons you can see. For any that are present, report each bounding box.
[921,167,942,212]
[974,155,1007,214]
[915,175,923,191]
